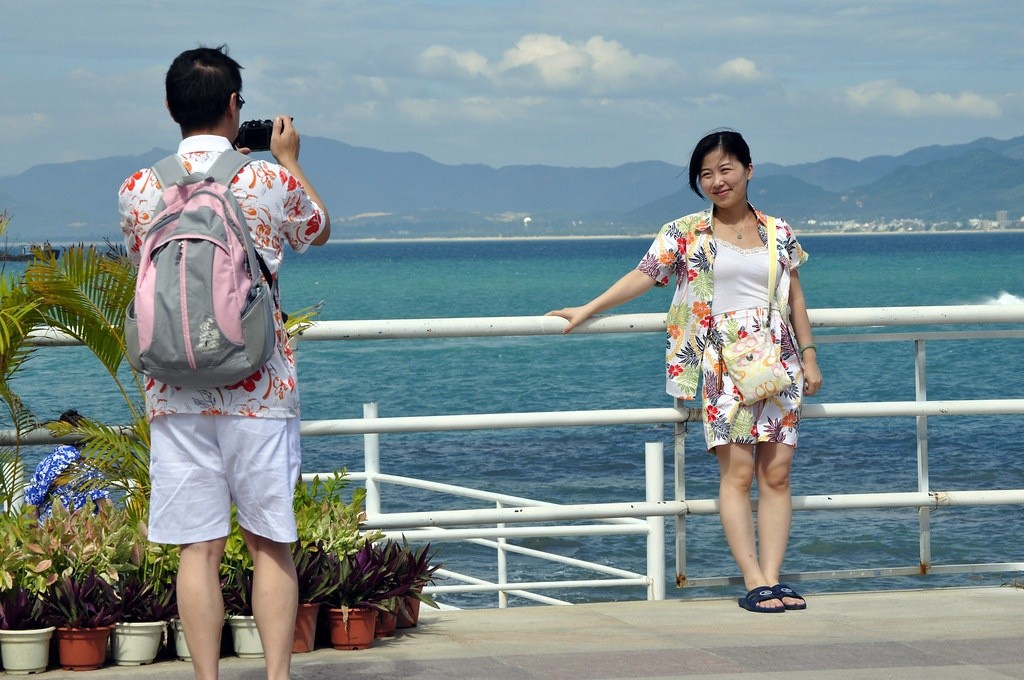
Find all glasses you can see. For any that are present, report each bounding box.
[232,90,245,110]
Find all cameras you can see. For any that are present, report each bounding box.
[238,119,285,153]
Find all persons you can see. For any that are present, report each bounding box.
[117,47,330,680]
[544,131,822,613]
[22,408,114,526]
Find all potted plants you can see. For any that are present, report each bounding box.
[0,207,450,675]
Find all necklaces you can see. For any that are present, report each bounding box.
[720,215,753,239]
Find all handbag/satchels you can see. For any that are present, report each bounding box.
[722,328,792,405]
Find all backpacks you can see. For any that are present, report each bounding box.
[125,149,275,388]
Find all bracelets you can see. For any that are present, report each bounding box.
[800,344,816,353]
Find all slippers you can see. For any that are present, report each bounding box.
[772,584,806,610]
[738,586,785,612]
